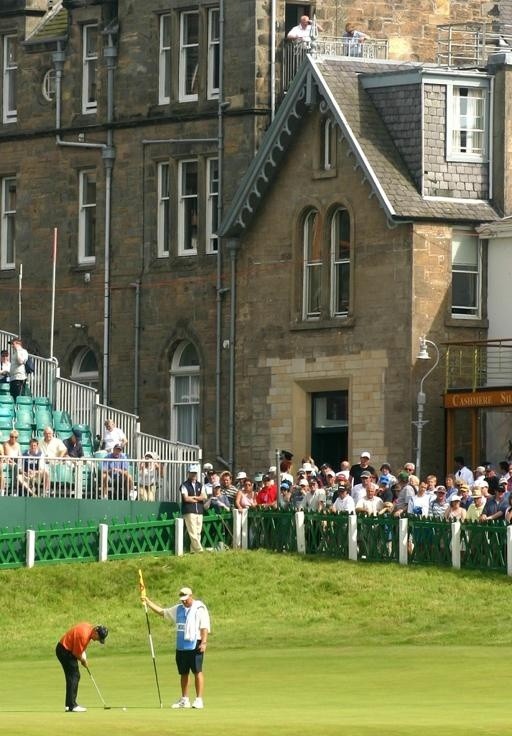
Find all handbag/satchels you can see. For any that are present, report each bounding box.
[25,355,34,374]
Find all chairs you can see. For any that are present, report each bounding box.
[0,380,139,498]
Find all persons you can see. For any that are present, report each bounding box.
[141,587,209,709]
[179,451,511,562]
[138,450,164,501]
[341,24,370,57]
[4,431,24,465]
[8,338,29,403]
[63,427,94,467]
[94,418,127,470]
[286,15,322,80]
[56,624,108,711]
[0,444,8,496]
[15,439,51,497]
[40,427,68,465]
[98,444,133,501]
[0,350,12,383]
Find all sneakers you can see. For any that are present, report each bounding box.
[65,706,69,711]
[72,706,87,712]
[192,698,204,708]
[172,698,191,708]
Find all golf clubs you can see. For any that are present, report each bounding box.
[86,666,112,709]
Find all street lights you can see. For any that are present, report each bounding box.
[410,337,440,479]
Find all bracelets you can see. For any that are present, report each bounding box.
[201,640,207,644]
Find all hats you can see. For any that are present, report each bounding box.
[361,451,371,460]
[203,462,214,470]
[399,472,409,480]
[7,338,22,345]
[145,451,154,459]
[279,463,347,492]
[114,444,122,451]
[213,481,221,489]
[474,466,486,472]
[486,463,496,471]
[177,587,193,600]
[359,470,372,478]
[187,465,198,473]
[73,429,82,440]
[435,477,508,501]
[236,471,247,480]
[96,625,108,645]
[262,474,270,480]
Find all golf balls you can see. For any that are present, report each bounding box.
[122,708,126,712]
